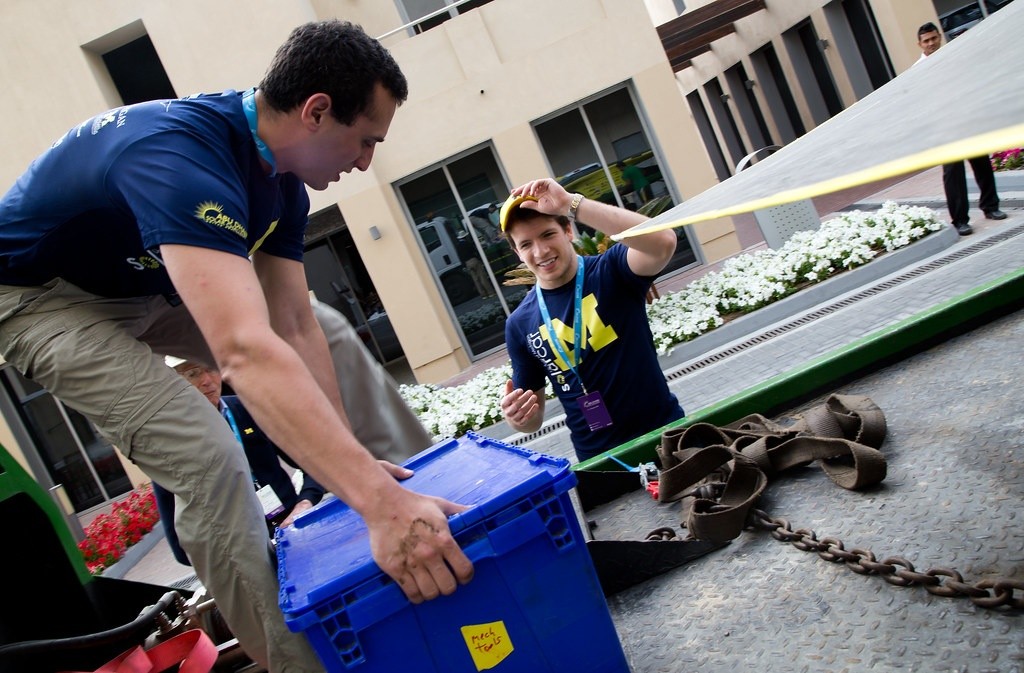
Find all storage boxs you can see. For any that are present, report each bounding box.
[275,429,631,672]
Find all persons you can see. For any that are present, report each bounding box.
[0,19,475,673]
[152,355,323,566]
[309,299,433,465]
[918,23,1006,236]
[501,178,685,463]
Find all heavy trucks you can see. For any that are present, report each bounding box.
[414,160,603,302]
[0,264,1024,673]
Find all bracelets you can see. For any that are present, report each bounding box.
[568,193,584,222]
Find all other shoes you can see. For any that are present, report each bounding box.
[985,209,1007,220]
[951,221,972,236]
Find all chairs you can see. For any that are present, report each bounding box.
[0,448,215,673]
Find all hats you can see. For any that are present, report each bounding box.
[165,354,187,368]
[499,184,538,233]
